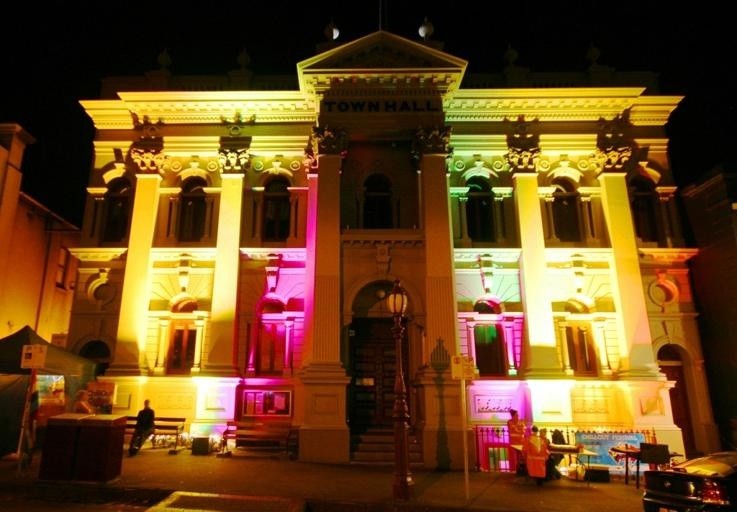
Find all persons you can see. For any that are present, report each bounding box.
[539,427,562,480]
[506,409,525,473]
[74,389,94,415]
[521,426,550,485]
[128,398,156,457]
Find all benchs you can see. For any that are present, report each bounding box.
[215,418,295,462]
[123,415,187,456]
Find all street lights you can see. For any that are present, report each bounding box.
[385,276,417,502]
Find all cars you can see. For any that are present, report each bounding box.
[639,450,736,510]
